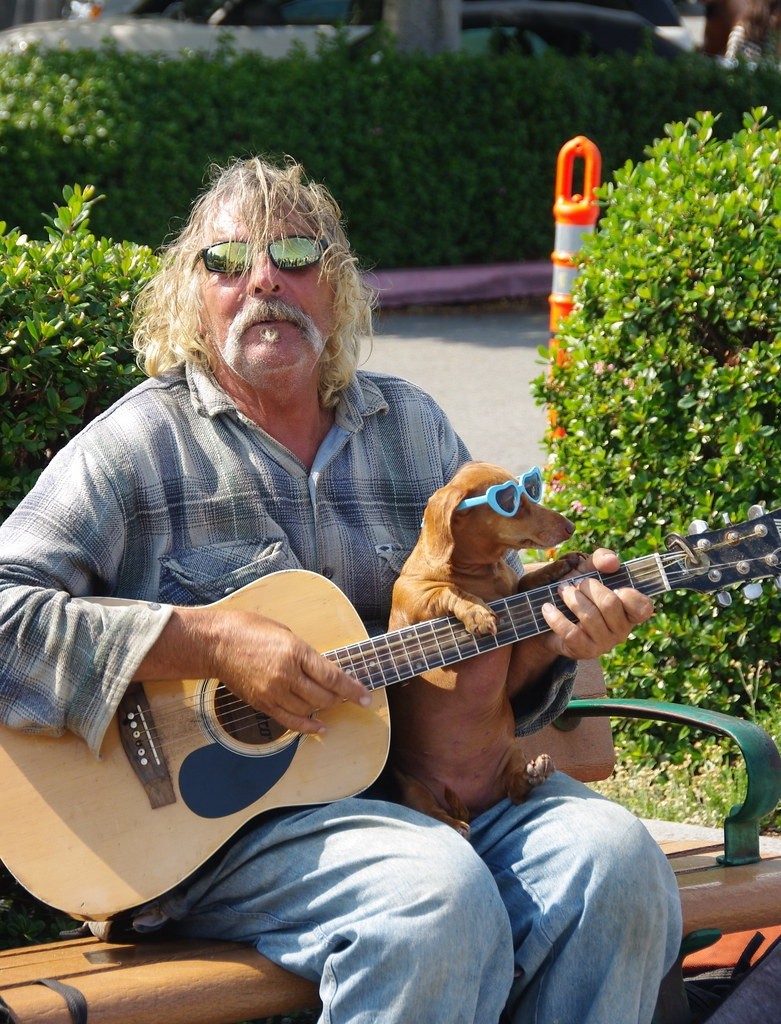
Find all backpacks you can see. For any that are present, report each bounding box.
[684,924,781,1024]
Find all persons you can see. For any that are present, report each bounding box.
[0,157,682,1024]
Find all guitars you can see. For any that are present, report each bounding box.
[1,505,781,927]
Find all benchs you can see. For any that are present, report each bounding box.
[0,653,781,1024]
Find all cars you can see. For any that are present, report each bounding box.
[0,0,699,68]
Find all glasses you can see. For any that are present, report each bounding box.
[191,235,329,273]
[454,466,543,518]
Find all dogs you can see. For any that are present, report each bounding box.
[383,461,589,828]
